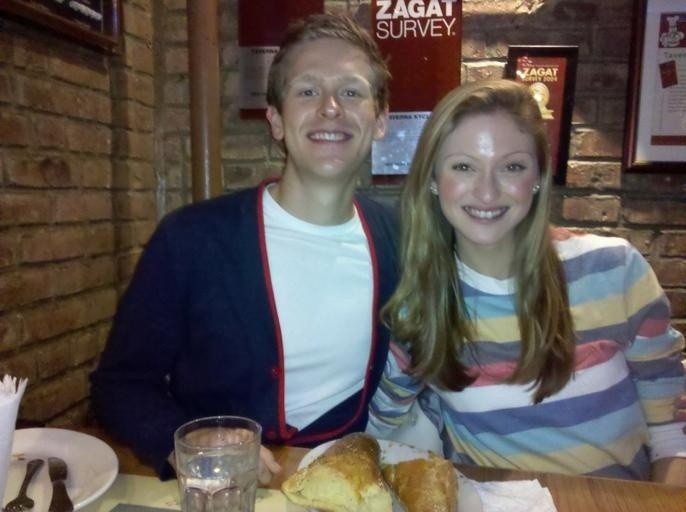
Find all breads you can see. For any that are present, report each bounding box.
[382,457,459,512]
[282,433,408,512]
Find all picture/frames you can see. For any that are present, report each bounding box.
[622,0,686,175]
[508,44,579,185]
[0,1,122,55]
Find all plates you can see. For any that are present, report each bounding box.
[297,438,482,510]
[0,428,119,512]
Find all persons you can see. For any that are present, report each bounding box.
[89,15,401,485]
[365,78,686,486]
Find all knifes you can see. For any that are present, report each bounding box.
[48,458,73,511]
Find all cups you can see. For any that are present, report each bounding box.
[174,414,262,511]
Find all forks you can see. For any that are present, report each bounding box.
[4,458,43,510]
